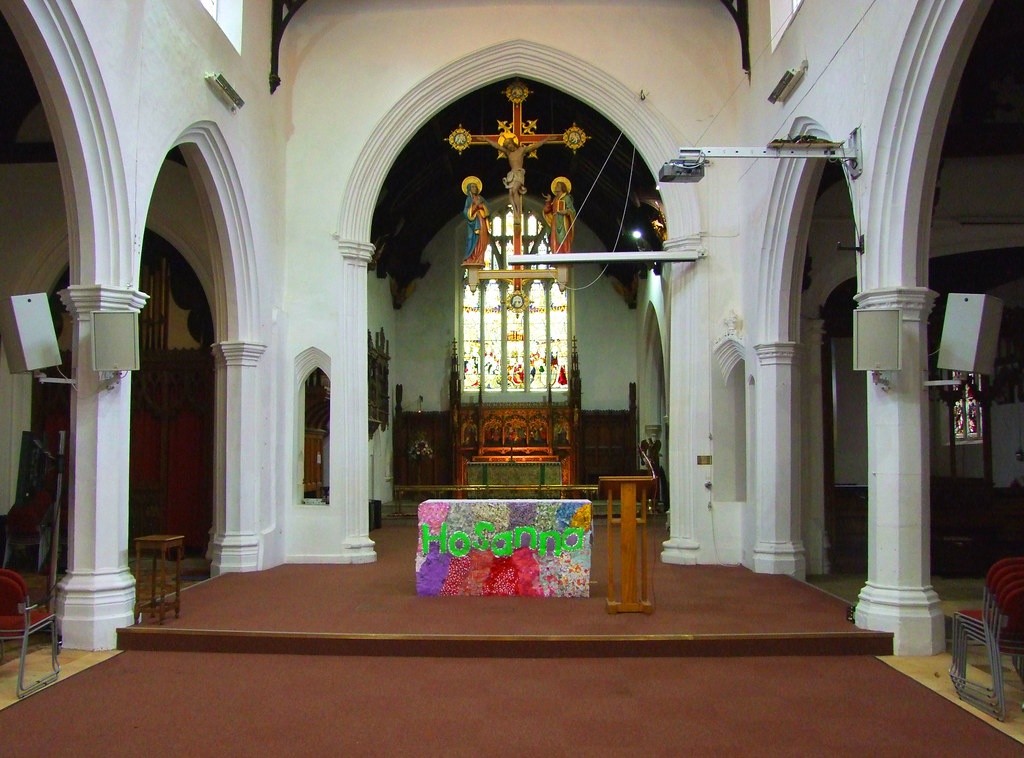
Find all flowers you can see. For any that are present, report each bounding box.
[406,430,434,466]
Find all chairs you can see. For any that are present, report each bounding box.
[949,557,1024,722]
[0,489,60,700]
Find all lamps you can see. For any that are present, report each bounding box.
[659,124,862,183]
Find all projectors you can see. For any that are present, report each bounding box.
[658,163,704,183]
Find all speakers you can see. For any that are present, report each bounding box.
[937,292,1004,376]
[381,243,420,288]
[90,310,140,371]
[0,293,63,374]
[852,308,902,371]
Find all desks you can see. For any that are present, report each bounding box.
[133,535,184,626]
[467,462,561,499]
[415,499,594,599]
